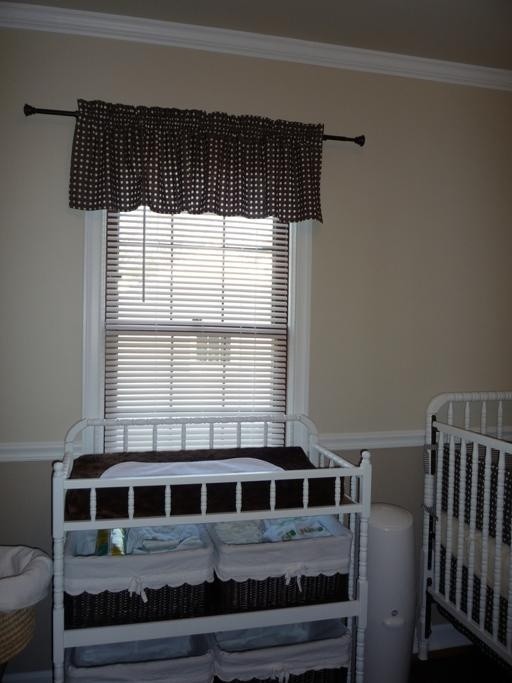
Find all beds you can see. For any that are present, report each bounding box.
[49,414,374,683]
[418,392,512,665]
